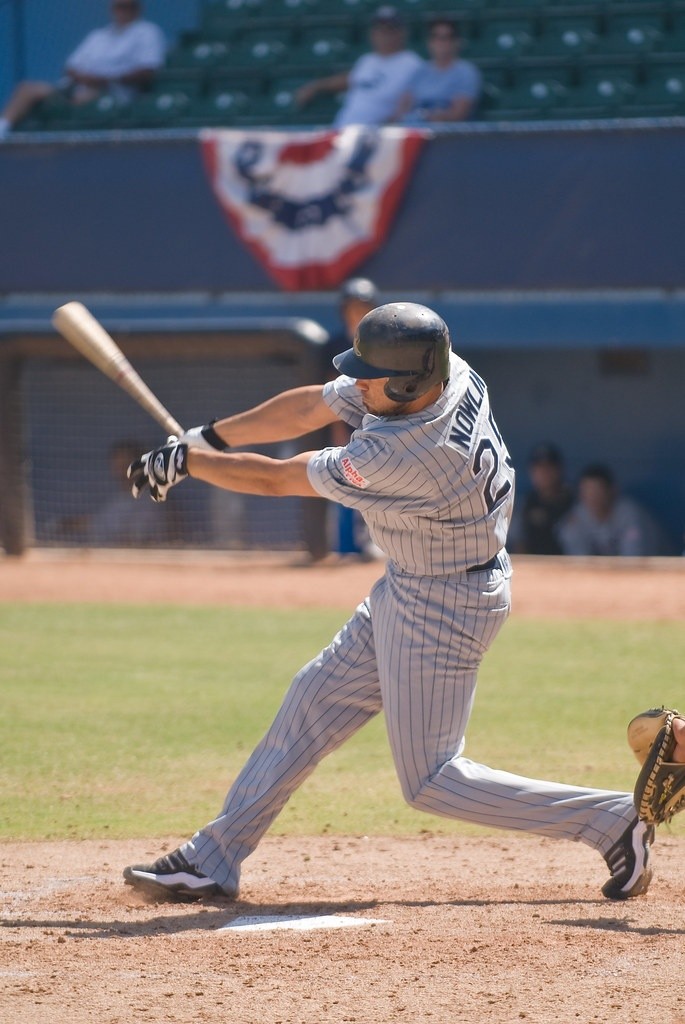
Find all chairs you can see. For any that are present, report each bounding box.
[9,0,685,135]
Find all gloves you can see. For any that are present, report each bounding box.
[126,434,189,503]
[181,420,228,452]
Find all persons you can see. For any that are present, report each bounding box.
[324,276,382,560]
[391,20,484,124]
[561,469,660,556]
[520,442,576,557]
[295,4,423,125]
[627,704,685,828]
[0,0,167,132]
[122,302,657,908]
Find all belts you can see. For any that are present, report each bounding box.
[466,555,501,572]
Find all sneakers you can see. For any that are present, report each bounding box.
[601,814,655,899]
[123,848,217,899]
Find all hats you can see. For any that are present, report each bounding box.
[370,6,399,21]
[343,277,377,302]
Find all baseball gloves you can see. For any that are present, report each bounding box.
[626,705,685,827]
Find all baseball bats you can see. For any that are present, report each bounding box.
[52,299,187,441]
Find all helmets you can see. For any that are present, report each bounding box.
[332,301,451,403]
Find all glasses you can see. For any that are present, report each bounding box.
[429,31,453,42]
